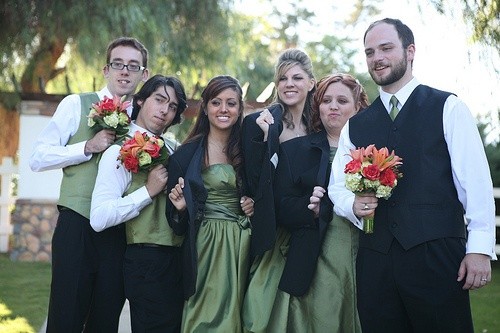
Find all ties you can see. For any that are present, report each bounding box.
[388,95,400,121]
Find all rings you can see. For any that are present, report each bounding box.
[481,279,487,281]
[365,203,368,210]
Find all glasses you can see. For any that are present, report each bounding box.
[107,62,145,72]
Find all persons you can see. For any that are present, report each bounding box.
[91,74,188,333]
[30,38,148,333]
[278,75,368,333]
[327,18,496,333]
[166,75,255,333]
[242,49,323,333]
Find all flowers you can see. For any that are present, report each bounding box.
[343,144,404,235]
[85,93,131,163]
[116,130,168,193]
